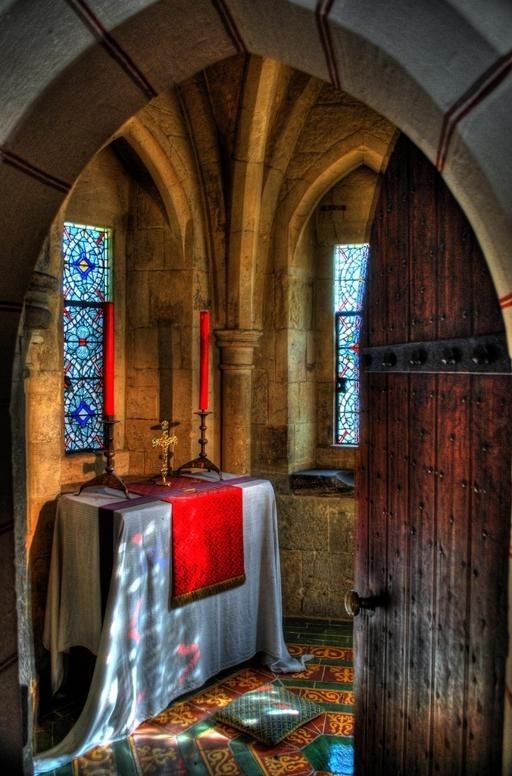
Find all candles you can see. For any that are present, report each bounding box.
[200,311,208,409]
[103,302,114,417]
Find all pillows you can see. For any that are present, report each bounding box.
[214,677,324,745]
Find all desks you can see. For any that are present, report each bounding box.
[32,471,314,775]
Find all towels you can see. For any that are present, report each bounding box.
[109,475,245,609]
